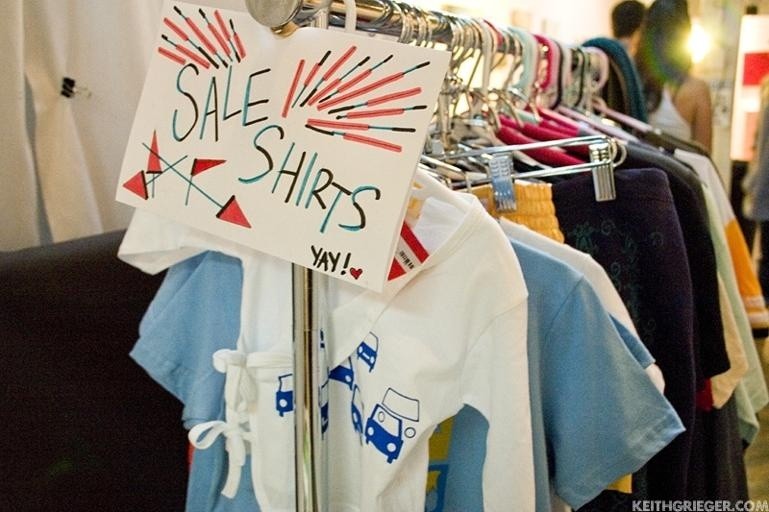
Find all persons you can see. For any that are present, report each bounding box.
[743,74,768,301]
[602,0,644,57]
[632,0,712,152]
